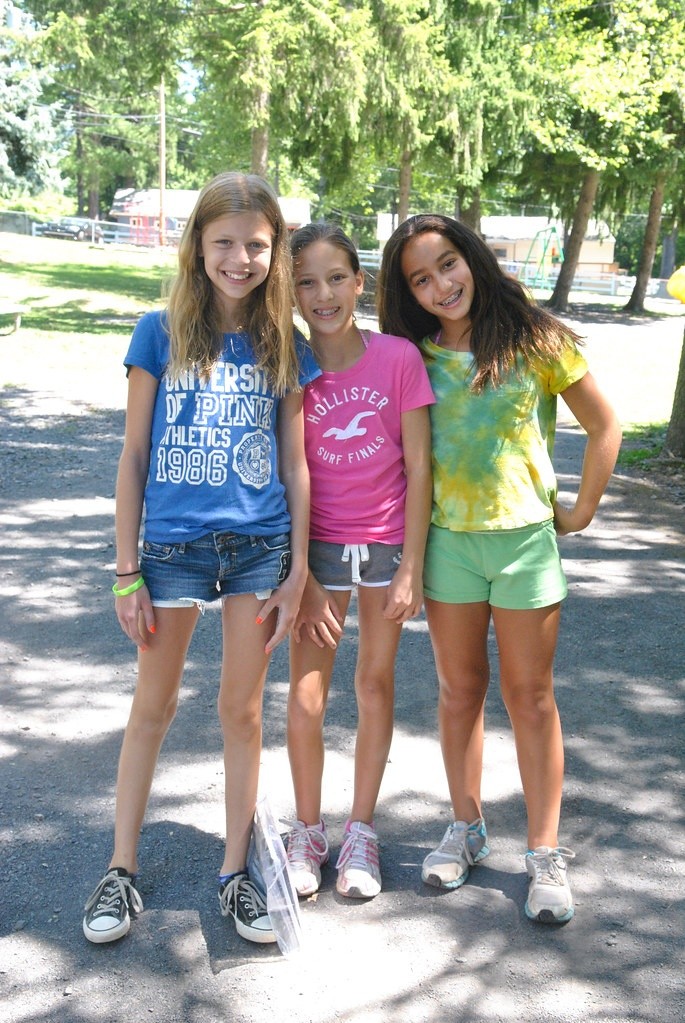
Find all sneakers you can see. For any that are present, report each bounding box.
[524,846,576,923]
[421,817,489,890]
[266,816,329,895]
[83,867,143,942]
[334,817,382,898]
[218,872,277,943]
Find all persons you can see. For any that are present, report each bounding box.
[81,172,316,945]
[285,220,438,901]
[375,212,623,926]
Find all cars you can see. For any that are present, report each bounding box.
[41,215,104,244]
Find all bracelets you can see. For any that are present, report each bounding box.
[116,570,142,578]
[112,577,146,597]
